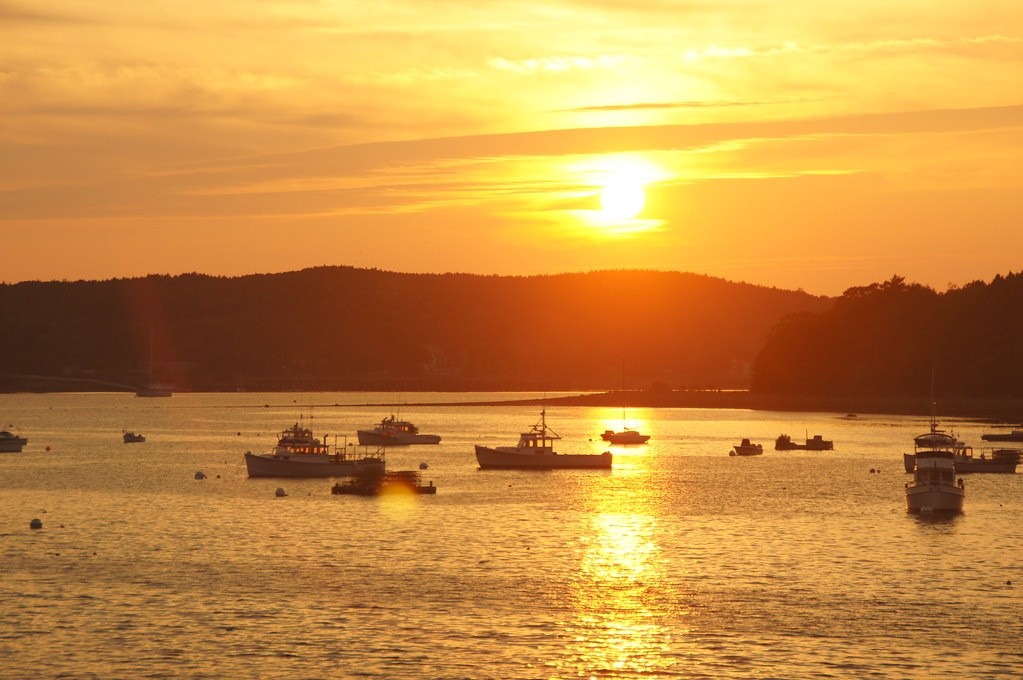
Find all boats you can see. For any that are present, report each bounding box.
[904,401,1019,510]
[475,392,612,469]
[123,427,145,443]
[137,327,173,397]
[357,396,442,445]
[243,406,436,495]
[600,360,650,444]
[774,430,833,450]
[733,438,763,455]
[0,431,27,452]
[981,429,1023,441]
